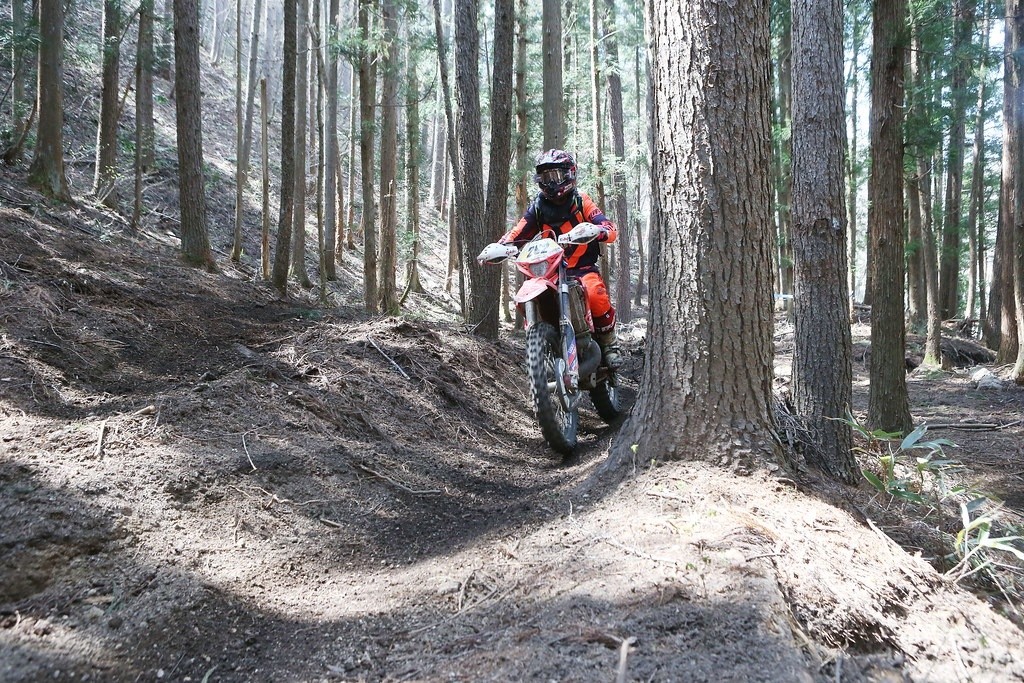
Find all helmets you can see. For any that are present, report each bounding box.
[532,148,576,202]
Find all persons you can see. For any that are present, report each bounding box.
[478,147,627,370]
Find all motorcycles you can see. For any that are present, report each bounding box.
[478,222,617,456]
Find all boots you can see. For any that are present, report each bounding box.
[597,329,623,369]
